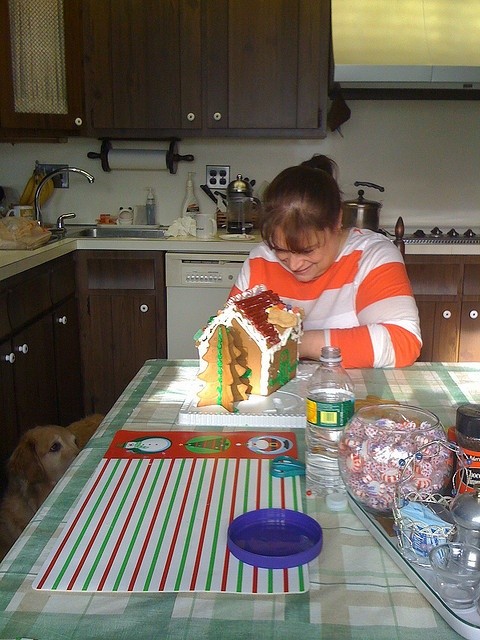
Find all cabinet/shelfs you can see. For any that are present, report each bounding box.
[0,2,82,136]
[78,250,166,421]
[402,254,480,362]
[55,251,83,428]
[1,263,54,491]
[83,0,328,140]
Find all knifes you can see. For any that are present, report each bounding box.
[200,185,228,214]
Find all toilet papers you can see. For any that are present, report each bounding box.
[107,147,170,171]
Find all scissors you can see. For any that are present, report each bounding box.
[268,454,307,479]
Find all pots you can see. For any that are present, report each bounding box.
[336,181,386,232]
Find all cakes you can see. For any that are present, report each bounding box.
[192,283,304,413]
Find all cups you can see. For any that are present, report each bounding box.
[430,544,480,611]
[195,214,217,239]
[6,206,33,221]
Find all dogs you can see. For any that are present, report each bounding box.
[0,412,104,564]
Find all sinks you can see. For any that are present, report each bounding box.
[66,227,169,241]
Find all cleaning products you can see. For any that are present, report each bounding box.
[181,171,200,219]
[144,187,157,224]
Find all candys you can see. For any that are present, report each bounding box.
[339,414,451,513]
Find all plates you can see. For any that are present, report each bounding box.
[219,234,255,241]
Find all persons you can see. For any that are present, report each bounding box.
[226,153,423,370]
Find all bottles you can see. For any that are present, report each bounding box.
[449,403,480,499]
[450,492,480,546]
[306,346,354,493]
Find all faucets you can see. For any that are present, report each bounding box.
[34,168,95,236]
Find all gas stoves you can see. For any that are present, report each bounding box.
[382,225,479,243]
